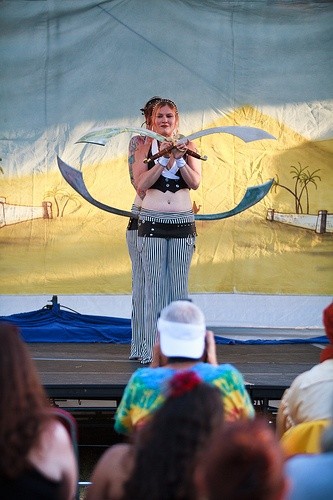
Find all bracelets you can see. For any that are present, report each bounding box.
[158,156,170,166]
[174,157,187,168]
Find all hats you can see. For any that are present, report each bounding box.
[157,300,206,359]
[319,303,333,363]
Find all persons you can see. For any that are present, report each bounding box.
[114,300,255,438]
[83,370,292,500]
[276,302,333,439]
[282,425,333,500]
[0,322,78,500]
[126,96,202,365]
